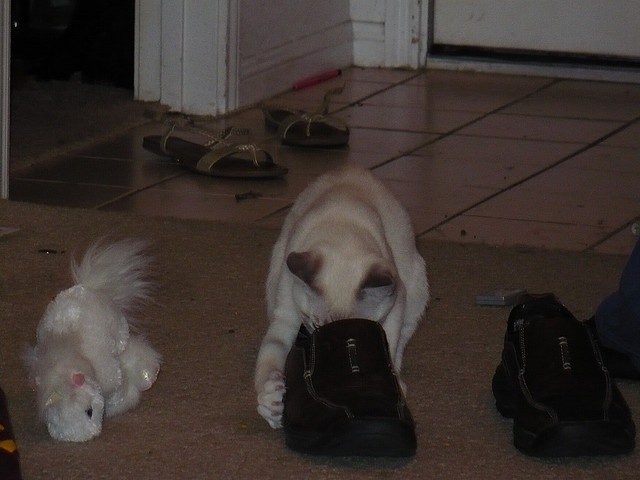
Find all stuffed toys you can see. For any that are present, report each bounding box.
[24,234,161,443]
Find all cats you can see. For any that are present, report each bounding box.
[254,162,430,430]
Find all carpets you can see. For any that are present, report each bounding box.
[7,77,170,172]
[0,200,640,480]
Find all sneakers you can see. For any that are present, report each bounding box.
[278,316,420,460]
[494,289,636,458]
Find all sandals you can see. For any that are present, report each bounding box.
[258,102,352,149]
[143,118,287,180]
